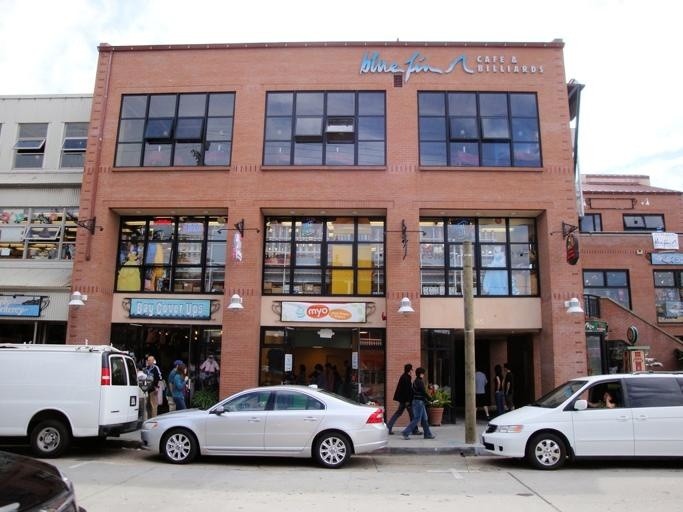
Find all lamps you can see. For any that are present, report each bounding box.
[228,294,243,309]
[68,292,85,305]
[398,298,414,312]
[565,298,584,313]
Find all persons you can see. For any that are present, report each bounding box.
[402,367,438,440]
[387,364,423,436]
[493,364,504,415]
[132,351,218,421]
[295,359,370,402]
[587,390,618,408]
[475,368,491,420]
[504,363,515,411]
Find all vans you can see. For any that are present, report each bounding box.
[480,371,683,470]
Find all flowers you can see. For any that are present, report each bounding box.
[427,384,451,407]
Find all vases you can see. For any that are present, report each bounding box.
[428,408,444,426]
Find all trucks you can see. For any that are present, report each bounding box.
[0,343,142,456]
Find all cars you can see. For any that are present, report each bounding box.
[193,362,295,393]
[0,450,87,511]
[140,385,388,467]
[361,385,382,403]
